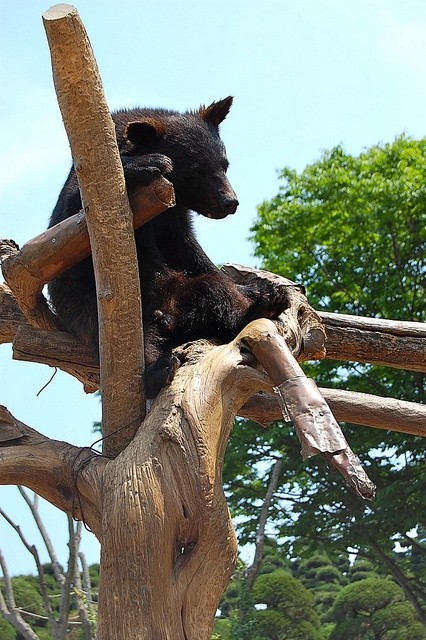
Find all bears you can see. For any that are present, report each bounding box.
[45,95,293,400]
